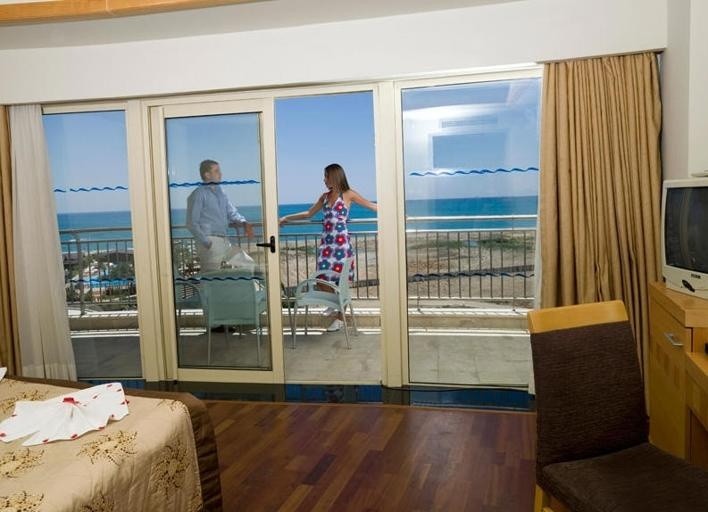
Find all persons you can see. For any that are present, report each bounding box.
[278,162,378,332]
[185,160,253,333]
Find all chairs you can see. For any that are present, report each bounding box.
[172,263,205,336]
[200,273,297,367]
[528,295,708,512]
[292,255,362,350]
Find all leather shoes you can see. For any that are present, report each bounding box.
[212,325,236,333]
[320,307,335,317]
[327,319,344,331]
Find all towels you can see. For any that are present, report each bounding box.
[0,380,131,449]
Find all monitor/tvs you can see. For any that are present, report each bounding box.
[660,178,708,300]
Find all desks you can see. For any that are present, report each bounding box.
[645,281,708,470]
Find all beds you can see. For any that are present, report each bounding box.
[0,372,225,511]
